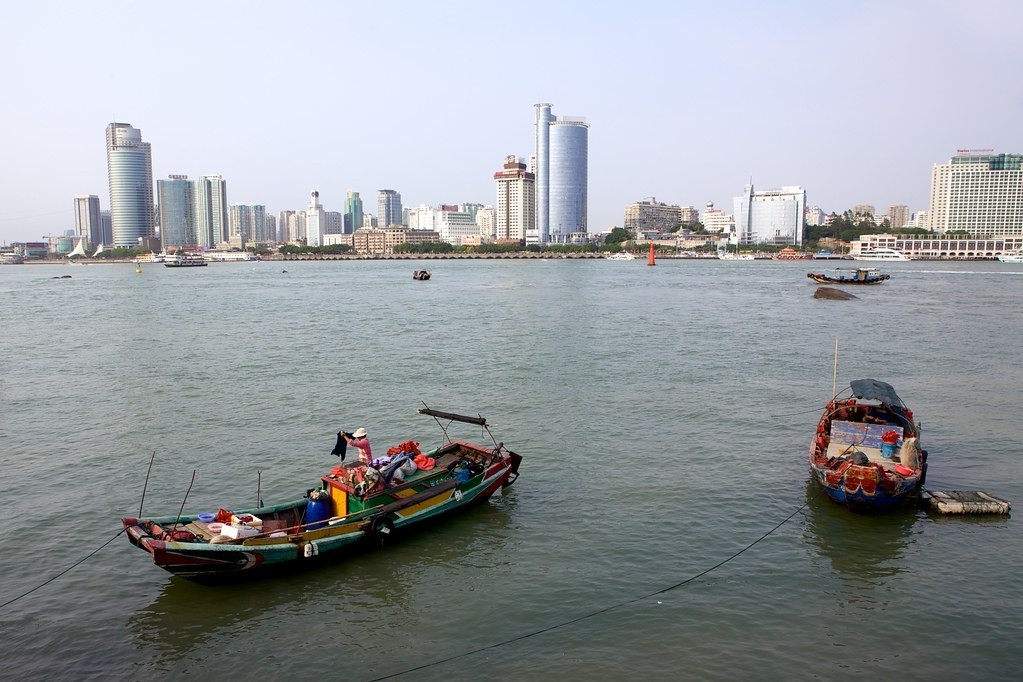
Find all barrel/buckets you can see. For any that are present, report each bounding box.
[305,497,332,531]
[882,443,897,459]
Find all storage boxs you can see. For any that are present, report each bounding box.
[221,514,287,539]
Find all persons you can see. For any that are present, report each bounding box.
[340,428,373,467]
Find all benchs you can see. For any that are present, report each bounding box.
[827,443,903,467]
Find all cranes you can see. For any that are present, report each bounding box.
[43,232,86,260]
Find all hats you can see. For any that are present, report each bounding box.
[352,427,368,438]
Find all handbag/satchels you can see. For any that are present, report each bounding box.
[216,508,231,522]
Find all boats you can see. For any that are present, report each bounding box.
[809,335,929,513]
[718,250,756,261]
[130,249,164,264]
[850,246,911,262]
[0,253,25,264]
[117,396,526,591]
[163,245,208,268]
[996,249,1023,264]
[412,266,432,280]
[806,266,892,286]
